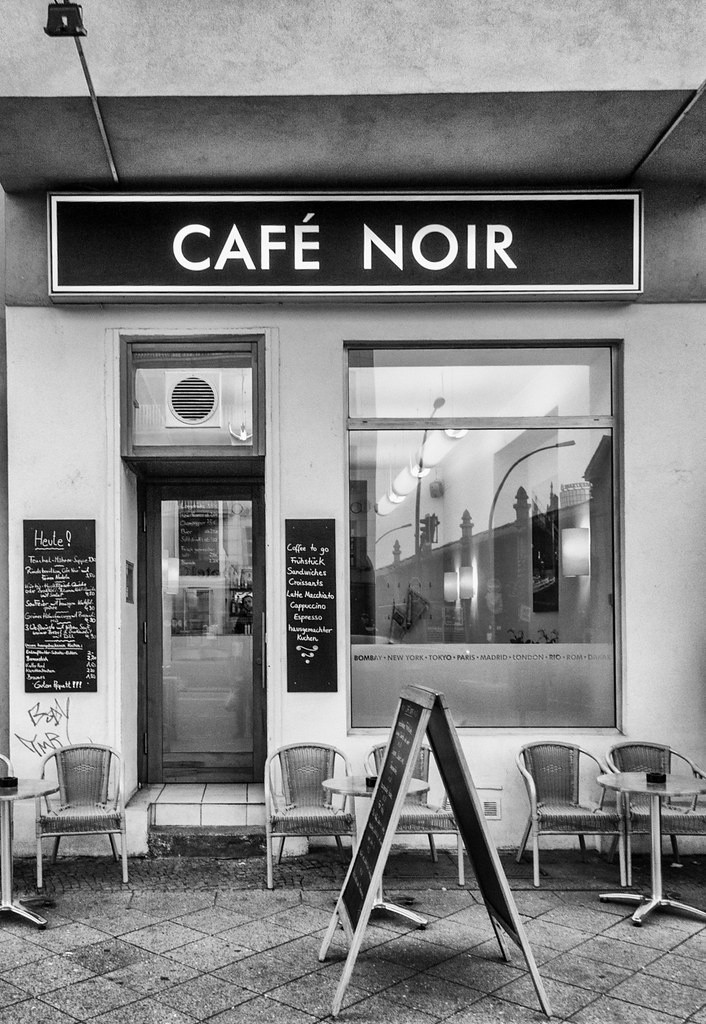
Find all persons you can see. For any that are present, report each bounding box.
[231,595,253,634]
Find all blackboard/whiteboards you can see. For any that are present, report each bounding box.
[23,519,98,691]
[285,518,339,693]
[337,683,436,954]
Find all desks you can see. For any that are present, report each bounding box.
[321,773,430,905]
[1,777,59,929]
[596,772,706,925]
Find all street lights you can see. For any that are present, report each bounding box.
[413,397,445,638]
[487,440,577,640]
[375,521,411,547]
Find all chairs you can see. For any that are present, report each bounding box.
[605,741,705,887]
[264,742,357,889]
[34,743,128,887]
[362,742,467,886]
[513,741,626,886]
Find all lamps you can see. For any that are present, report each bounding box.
[560,527,588,577]
[391,465,430,496]
[373,491,405,517]
[458,566,473,600]
[415,430,467,467]
[443,572,457,602]
